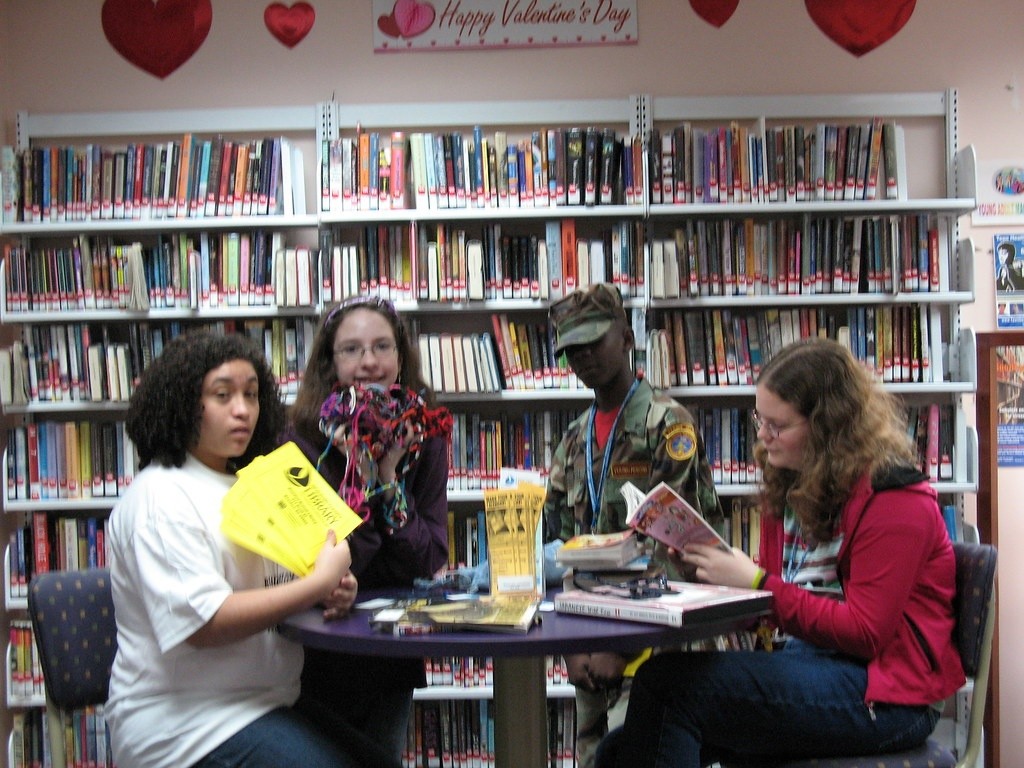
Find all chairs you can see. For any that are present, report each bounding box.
[718,541,998,768]
[27,567,118,768]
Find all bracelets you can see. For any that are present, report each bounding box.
[757,572,769,589]
[751,566,764,589]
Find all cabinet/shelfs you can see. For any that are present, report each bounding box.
[330,93,641,768]
[641,86,985,768]
[0,102,328,768]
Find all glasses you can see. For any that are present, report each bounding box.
[750,407,811,439]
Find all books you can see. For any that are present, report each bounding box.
[0,120,954,768]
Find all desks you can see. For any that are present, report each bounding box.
[276,589,756,768]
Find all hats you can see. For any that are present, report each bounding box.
[546,281,623,359]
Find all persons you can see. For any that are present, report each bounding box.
[541,284,725,768]
[101,330,358,768]
[277,292,451,768]
[593,337,971,768]
